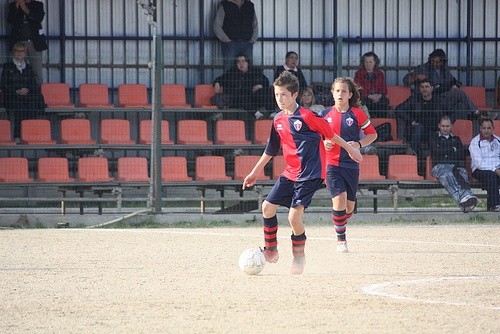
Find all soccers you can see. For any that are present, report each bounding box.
[238,249,266,276]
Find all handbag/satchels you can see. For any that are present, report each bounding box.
[310,82,335,106]
[34,34,47,52]
[210,94,233,109]
[372,122,393,143]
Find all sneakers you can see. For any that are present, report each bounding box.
[259,246,279,264]
[289,257,306,275]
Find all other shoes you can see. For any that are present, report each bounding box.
[459,195,478,207]
[489,205,500,211]
[336,241,349,253]
[464,202,477,213]
[14,138,21,144]
[477,112,499,126]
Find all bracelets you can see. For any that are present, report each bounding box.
[357,140,362,149]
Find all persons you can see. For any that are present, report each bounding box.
[211,0,498,179]
[318,75,378,252]
[242,71,364,273]
[0,0,50,144]
[468,119,500,212]
[430,114,479,213]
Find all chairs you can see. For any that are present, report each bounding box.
[0,84,500,215]
